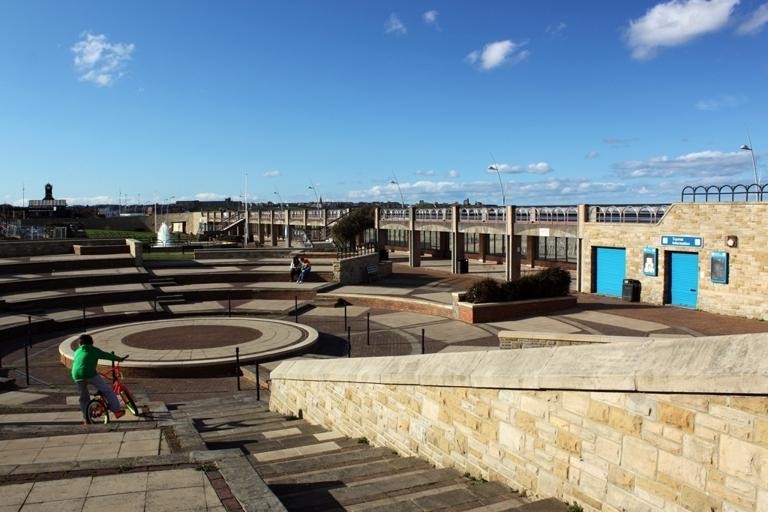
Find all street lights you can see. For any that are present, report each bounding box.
[741,143,760,203]
[239,166,252,248]
[116,189,176,214]
[392,180,405,209]
[488,166,506,206]
[306,185,320,218]
[274,190,283,213]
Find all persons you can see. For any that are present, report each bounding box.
[289,255,301,283]
[69,335,131,425]
[296,257,311,284]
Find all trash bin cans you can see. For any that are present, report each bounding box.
[622,279,640,302]
[457,259,468,273]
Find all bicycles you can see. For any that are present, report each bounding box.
[84,352,138,427]
